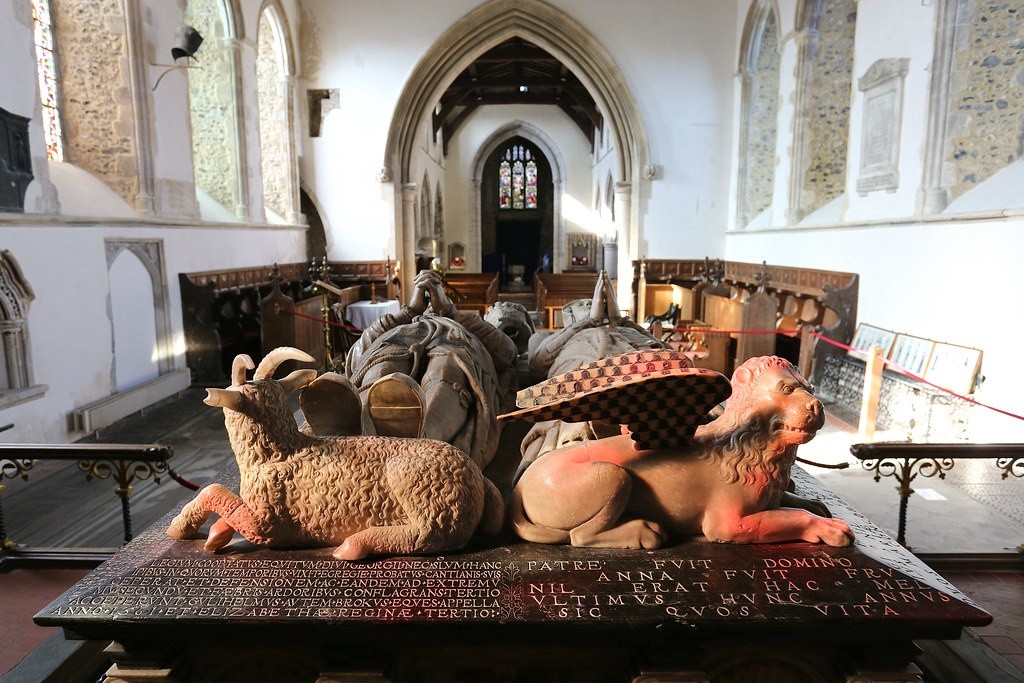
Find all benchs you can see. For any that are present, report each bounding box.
[439,270,500,318]
[533,271,608,330]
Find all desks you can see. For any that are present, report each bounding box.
[345,298,401,334]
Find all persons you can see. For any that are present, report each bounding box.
[484,301,536,354]
[299,269,518,473]
[512,270,725,486]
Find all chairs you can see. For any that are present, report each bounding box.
[330,301,366,365]
[635,249,858,384]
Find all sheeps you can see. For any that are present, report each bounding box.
[165,347,504,561]
